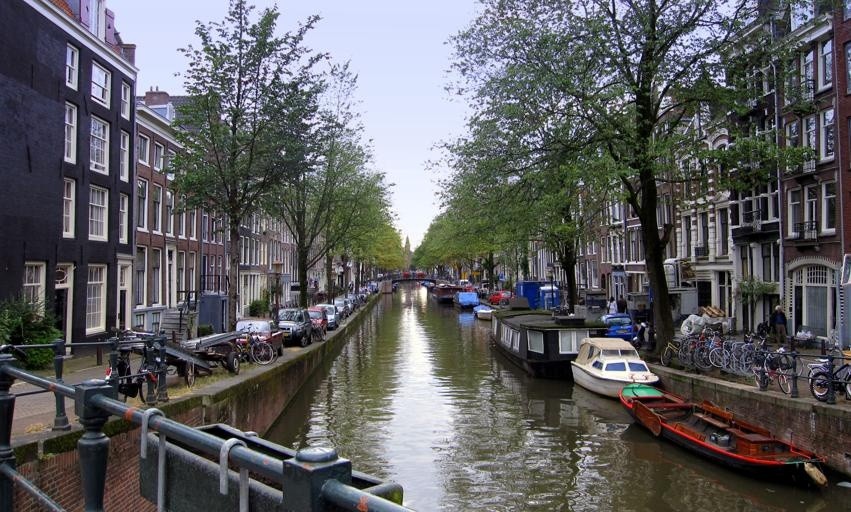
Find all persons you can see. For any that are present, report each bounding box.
[467,276,472,285]
[349,281,354,294]
[606,294,629,315]
[769,305,788,353]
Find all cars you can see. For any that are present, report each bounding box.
[221,270,411,366]
[596,313,639,342]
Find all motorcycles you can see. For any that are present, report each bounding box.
[631,318,658,352]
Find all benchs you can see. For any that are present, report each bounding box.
[784,326,818,349]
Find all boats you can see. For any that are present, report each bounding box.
[489,345,581,428]
[571,337,661,399]
[619,382,826,486]
[620,421,816,511]
[417,277,516,323]
[488,294,609,380]
[572,383,637,425]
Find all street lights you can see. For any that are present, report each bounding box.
[479,265,483,282]
[547,267,557,307]
[464,269,467,284]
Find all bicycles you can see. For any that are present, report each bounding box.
[105,327,162,406]
[752,351,792,394]
[661,323,805,380]
[807,344,851,397]
[810,358,851,402]
